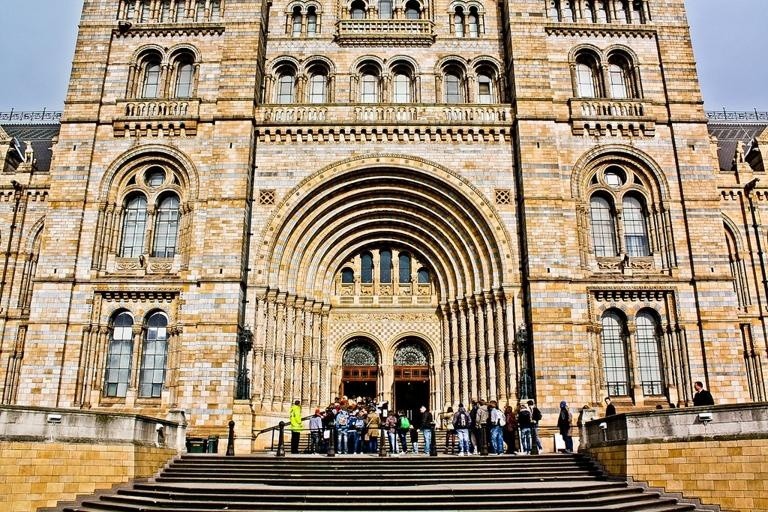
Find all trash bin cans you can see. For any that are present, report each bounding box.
[188,434,219,452]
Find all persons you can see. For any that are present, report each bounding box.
[604,397,616,417]
[290,395,433,454]
[693,382,715,406]
[556,402,573,452]
[578,404,589,421]
[438,398,542,455]
